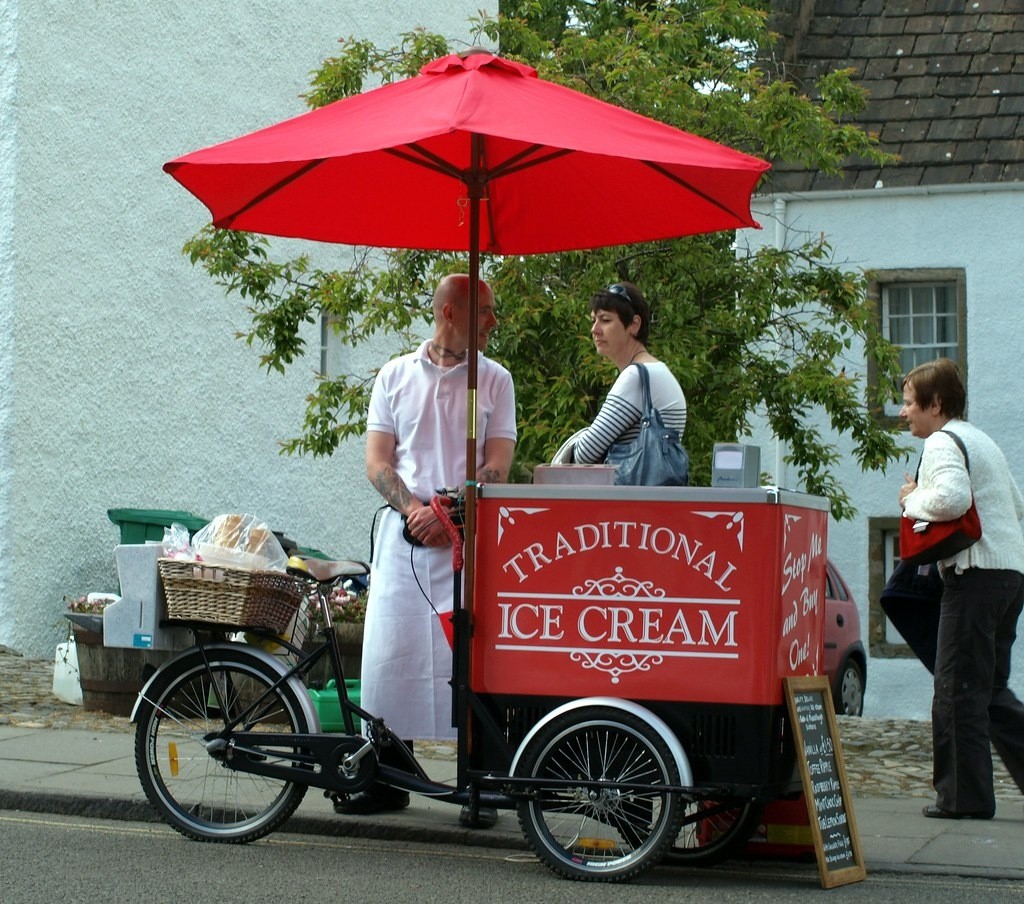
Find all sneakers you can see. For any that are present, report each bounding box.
[459,805,497,829]
[334,786,409,812]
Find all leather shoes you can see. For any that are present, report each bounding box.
[922,804,995,819]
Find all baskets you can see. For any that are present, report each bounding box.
[158,558,309,636]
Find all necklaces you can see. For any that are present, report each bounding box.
[628,349,648,364]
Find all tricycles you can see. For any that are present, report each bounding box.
[128,484,832,885]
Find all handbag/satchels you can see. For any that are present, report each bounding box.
[401,498,469,547]
[601,363,688,486]
[900,430,982,569]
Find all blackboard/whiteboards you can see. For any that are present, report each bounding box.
[784,678,871,887]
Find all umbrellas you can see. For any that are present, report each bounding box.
[163,50,771,750]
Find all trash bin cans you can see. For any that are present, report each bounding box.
[107,506,213,596]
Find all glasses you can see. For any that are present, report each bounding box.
[603,284,630,300]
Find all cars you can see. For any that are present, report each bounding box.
[820,557,868,717]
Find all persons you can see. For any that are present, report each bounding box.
[553,282,687,488]
[898,358,1024,820]
[331,273,518,827]
